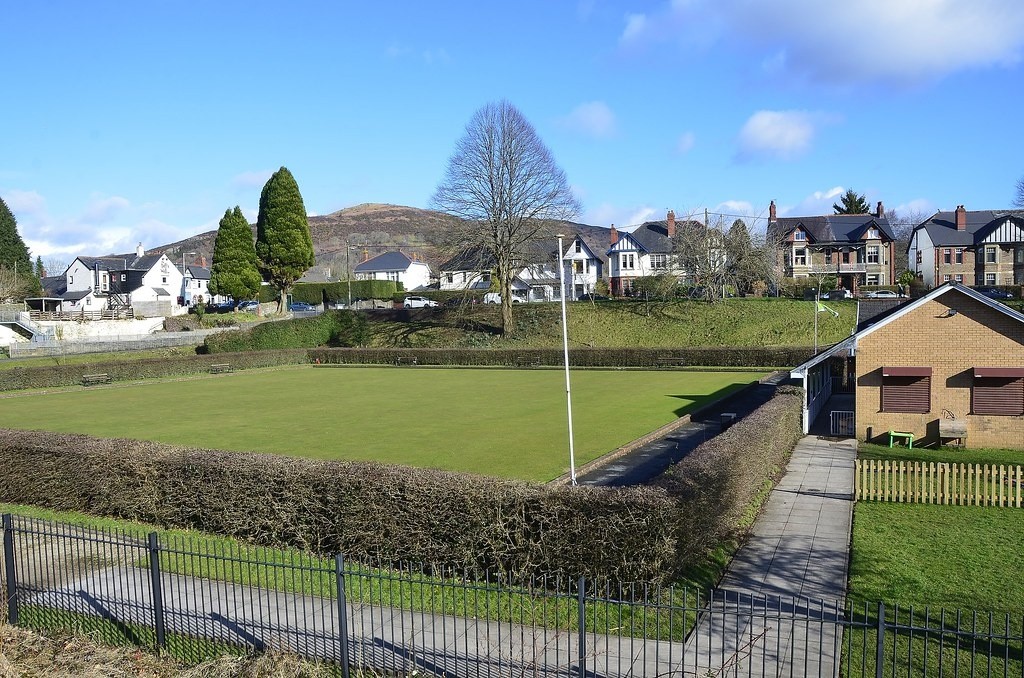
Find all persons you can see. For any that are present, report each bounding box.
[624,285,640,297]
[897,283,905,298]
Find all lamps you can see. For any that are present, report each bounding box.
[949,308,956,315]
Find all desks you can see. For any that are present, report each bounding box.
[887,430,914,449]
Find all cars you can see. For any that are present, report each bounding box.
[484,291,524,305]
[689,285,733,298]
[188,299,258,315]
[864,289,902,299]
[403,296,439,308]
[578,293,609,301]
[805,288,852,299]
[289,301,315,311]
[443,293,480,306]
[974,285,1013,301]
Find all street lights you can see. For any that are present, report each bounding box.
[182,252,197,276]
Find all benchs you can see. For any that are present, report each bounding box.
[81,373,112,386]
[211,362,233,375]
[396,357,418,367]
[655,357,683,367]
[516,357,540,369]
